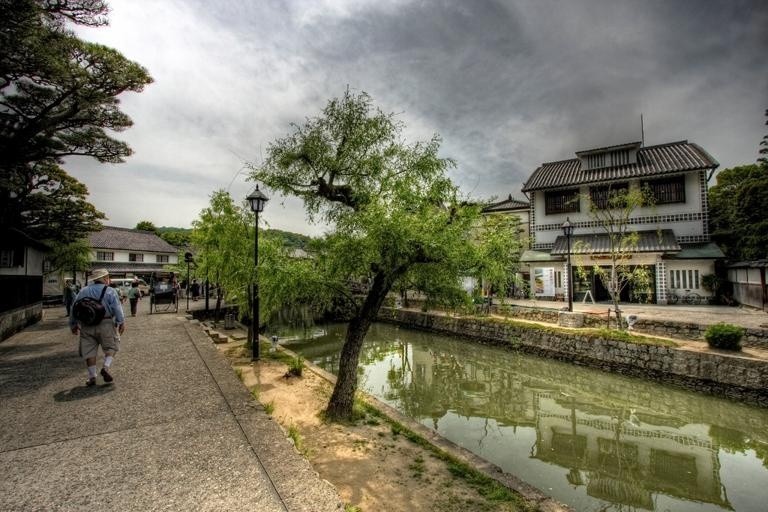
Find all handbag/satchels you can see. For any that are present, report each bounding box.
[134,292,140,297]
[72,296,105,326]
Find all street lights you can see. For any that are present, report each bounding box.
[246,184,269,361]
[561,217,576,312]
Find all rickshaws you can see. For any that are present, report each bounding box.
[150,271,179,314]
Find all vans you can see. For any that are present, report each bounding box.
[110,279,152,297]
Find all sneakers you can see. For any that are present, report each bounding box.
[85,377,96,386]
[101,366,113,382]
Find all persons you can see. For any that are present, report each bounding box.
[190,279,200,301]
[109,279,152,316]
[62,279,76,317]
[156,278,170,294]
[210,284,215,296]
[70,269,126,387]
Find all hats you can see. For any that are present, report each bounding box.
[87,268,110,282]
